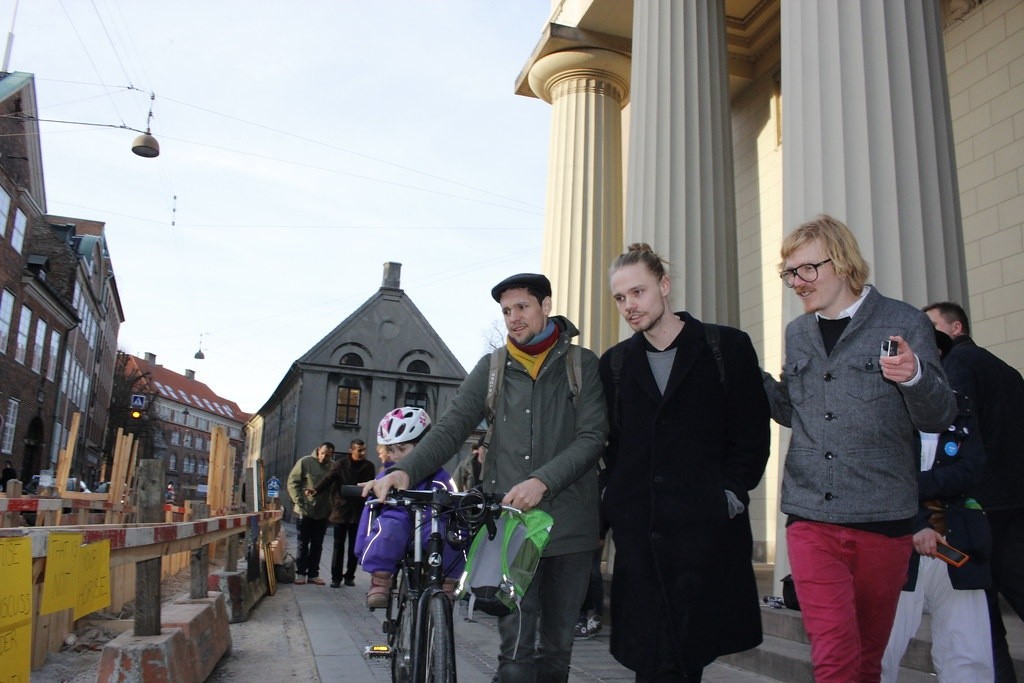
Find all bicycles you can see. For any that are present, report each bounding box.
[342,484,522,683]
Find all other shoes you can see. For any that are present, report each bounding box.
[295,574,306,584]
[308,577,324,586]
[344,577,354,586]
[329,580,340,586]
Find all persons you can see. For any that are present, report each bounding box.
[574,446,611,640]
[354,407,469,609]
[0,461,17,492]
[287,442,336,585]
[760,215,957,683]
[304,439,375,588]
[376,445,389,471]
[452,435,485,492]
[599,244,770,683]
[357,273,609,683]
[889,302,1024,683]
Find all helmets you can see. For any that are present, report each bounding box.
[376,407,432,445]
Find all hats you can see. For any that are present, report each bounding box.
[491,273,552,303]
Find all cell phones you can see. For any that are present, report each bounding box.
[936,543,969,567]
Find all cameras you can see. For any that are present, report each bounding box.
[880,340,898,357]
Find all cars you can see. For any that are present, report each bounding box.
[91,481,127,512]
[21,475,75,518]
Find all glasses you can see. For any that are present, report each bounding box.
[779,257,831,287]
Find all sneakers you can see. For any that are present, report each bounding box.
[439,580,456,608]
[574,618,589,641]
[366,571,394,608]
[586,615,603,638]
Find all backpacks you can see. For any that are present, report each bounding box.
[458,505,554,616]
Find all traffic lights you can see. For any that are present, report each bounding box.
[130,408,142,422]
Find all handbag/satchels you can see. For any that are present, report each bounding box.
[273,553,295,582]
